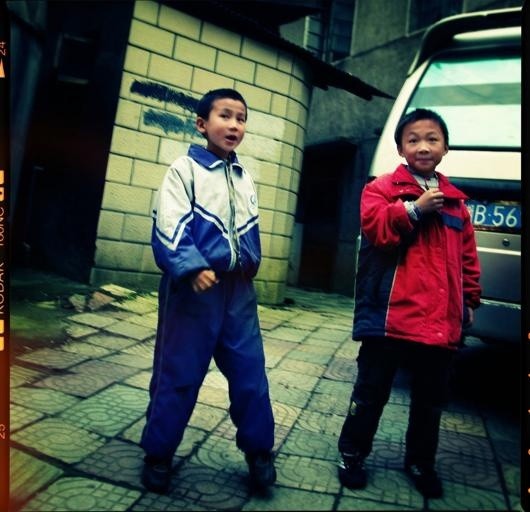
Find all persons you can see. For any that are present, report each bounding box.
[137,88,276,497]
[334,107,483,497]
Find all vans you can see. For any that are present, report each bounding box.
[358,3,530,381]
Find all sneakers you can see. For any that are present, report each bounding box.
[143,455,172,492]
[337,451,367,488]
[245,448,276,483]
[404,462,443,497]
